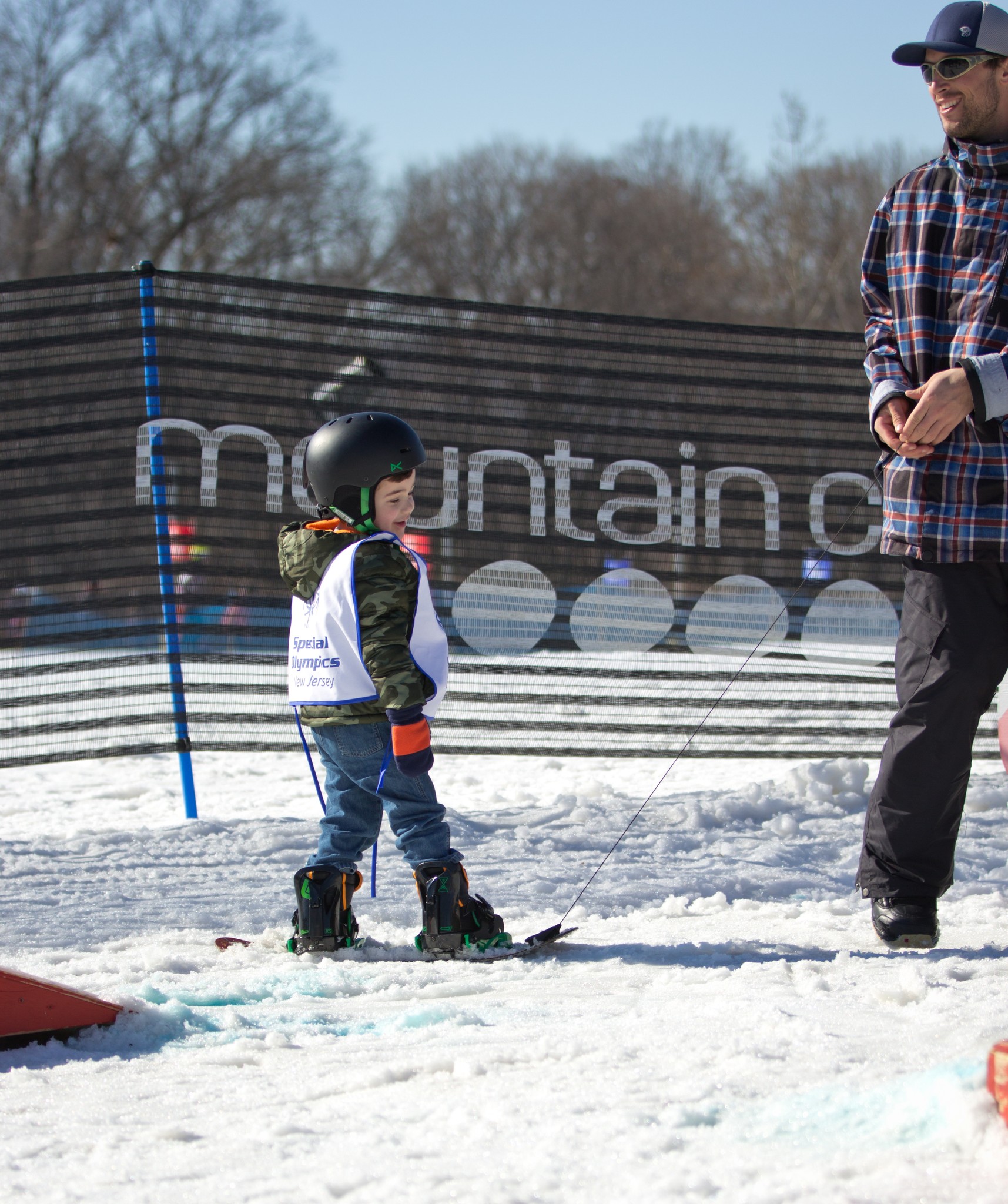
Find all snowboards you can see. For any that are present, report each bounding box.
[214,924,580,962]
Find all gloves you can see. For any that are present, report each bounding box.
[386,703,434,779]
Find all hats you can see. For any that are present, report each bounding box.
[892,1,1008,67]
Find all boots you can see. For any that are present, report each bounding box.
[286,865,366,954]
[413,861,512,958]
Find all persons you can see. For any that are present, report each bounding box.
[852,0,1008,950]
[277,412,512,954]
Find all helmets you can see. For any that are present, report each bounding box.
[303,413,427,507]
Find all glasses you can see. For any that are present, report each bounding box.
[921,54,999,85]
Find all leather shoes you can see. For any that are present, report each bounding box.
[872,895,939,949]
